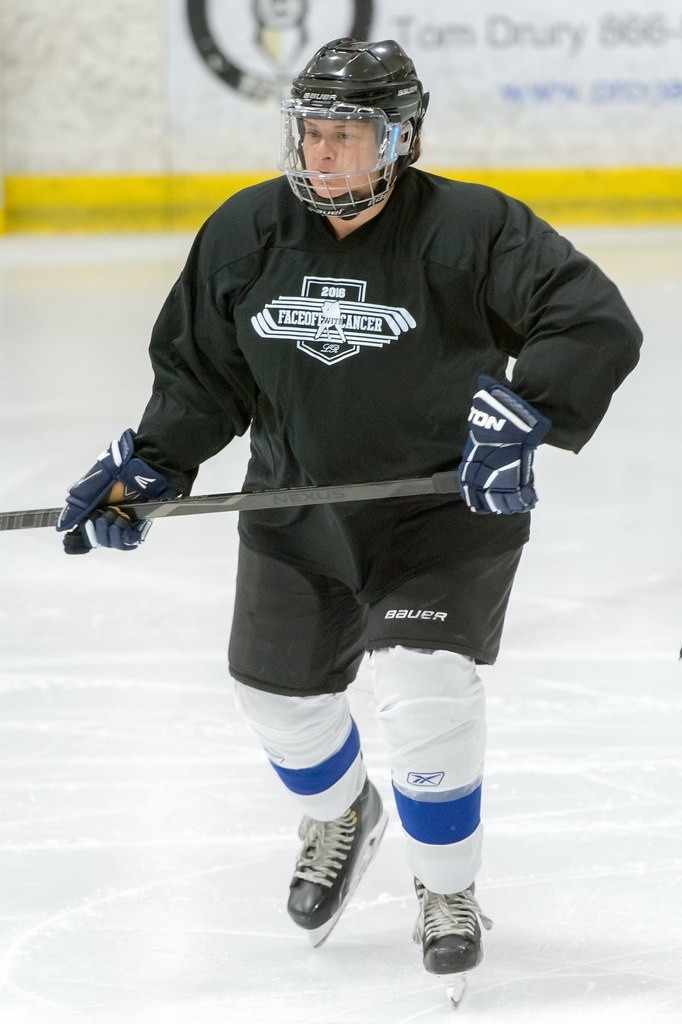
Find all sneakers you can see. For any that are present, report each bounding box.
[287,776,388,949]
[414,877,491,1006]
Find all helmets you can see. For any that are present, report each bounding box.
[290,38,430,221]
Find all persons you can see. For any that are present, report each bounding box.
[55,37,643,1006]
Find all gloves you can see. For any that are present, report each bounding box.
[458,374,551,515]
[56,429,182,554]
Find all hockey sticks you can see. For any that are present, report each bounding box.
[0,470,460,531]
